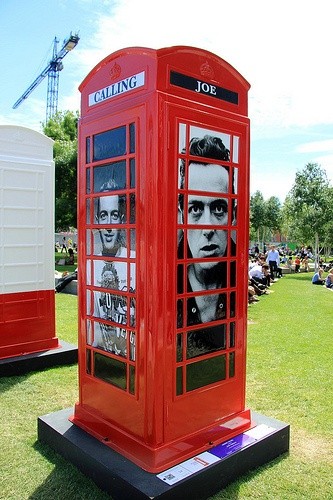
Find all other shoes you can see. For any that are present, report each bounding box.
[249,297,260,301]
[270,280,276,283]
[265,292,269,295]
[259,290,266,296]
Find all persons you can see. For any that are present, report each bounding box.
[246,239,332,304]
[179,135,238,352]
[55,236,77,265]
[84,179,138,360]
[55,266,78,294]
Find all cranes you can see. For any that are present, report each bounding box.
[11,30,80,127]
